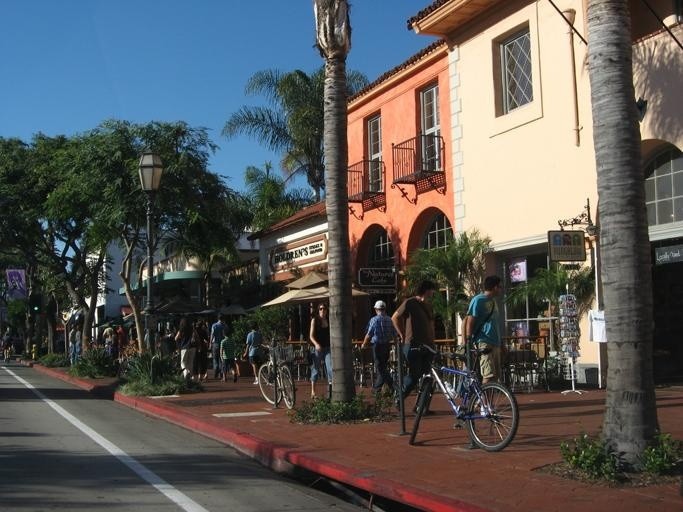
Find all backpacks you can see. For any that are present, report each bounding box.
[190,329,200,347]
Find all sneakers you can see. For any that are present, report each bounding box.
[480,405,496,416]
[183,368,238,383]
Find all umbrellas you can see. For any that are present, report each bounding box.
[259,269,371,319]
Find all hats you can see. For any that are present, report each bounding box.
[374,300,386,309]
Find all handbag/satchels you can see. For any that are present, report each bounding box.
[461,316,467,345]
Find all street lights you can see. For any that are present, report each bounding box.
[138,148,163,361]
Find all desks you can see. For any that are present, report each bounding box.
[502,350,536,393]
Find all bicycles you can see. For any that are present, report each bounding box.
[109,350,134,380]
[409,342,520,453]
[252,337,297,409]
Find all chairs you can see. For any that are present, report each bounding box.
[350,357,545,393]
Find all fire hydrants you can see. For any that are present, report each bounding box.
[31,344,38,360]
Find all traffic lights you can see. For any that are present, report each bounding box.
[30,294,42,313]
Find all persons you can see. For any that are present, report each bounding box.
[360,299,397,396]
[460,315,468,373]
[309,303,332,398]
[1,333,11,362]
[242,322,262,384]
[68,314,237,384]
[392,278,436,413]
[468,274,500,415]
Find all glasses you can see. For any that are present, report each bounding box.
[319,308,326,310]
